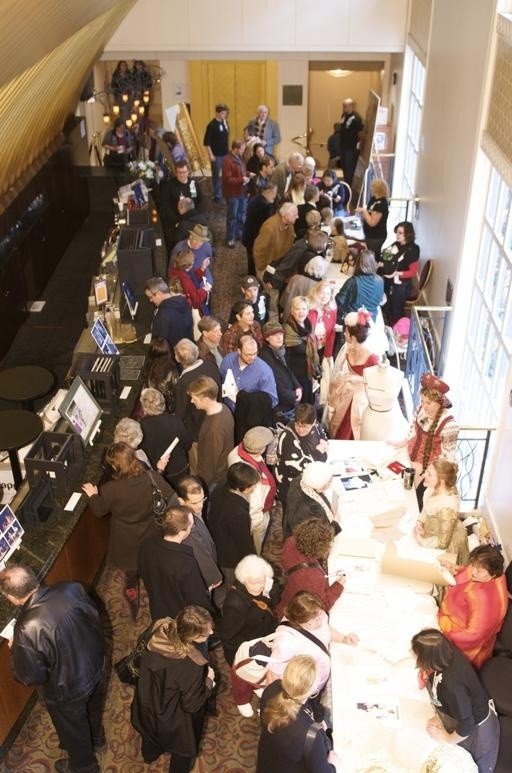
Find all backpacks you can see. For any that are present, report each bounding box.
[162,374,176,414]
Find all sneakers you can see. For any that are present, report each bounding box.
[54,759,100,773]
[253,688,264,698]
[215,196,220,202]
[227,239,234,248]
[237,702,254,718]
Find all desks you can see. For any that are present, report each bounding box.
[0,407,43,492]
[0,363,59,412]
[323,435,458,773]
[321,216,386,333]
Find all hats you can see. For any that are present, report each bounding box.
[420,374,449,395]
[243,426,273,454]
[188,223,210,241]
[241,275,258,290]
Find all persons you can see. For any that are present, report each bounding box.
[111,60,131,97]
[414,459,470,567]
[394,372,461,513]
[103,96,422,443]
[412,629,500,772]
[437,544,508,672]
[129,60,153,96]
[82,297,339,772]
[2,564,112,773]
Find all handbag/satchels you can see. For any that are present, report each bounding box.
[192,308,202,342]
[114,622,162,685]
[233,635,272,687]
[265,422,285,466]
[104,154,125,168]
[152,491,167,528]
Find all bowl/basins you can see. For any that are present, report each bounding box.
[418,373,450,394]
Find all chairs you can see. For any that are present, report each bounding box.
[403,258,435,310]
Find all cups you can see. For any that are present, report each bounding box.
[400,467,417,489]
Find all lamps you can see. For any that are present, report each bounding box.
[87,64,168,130]
